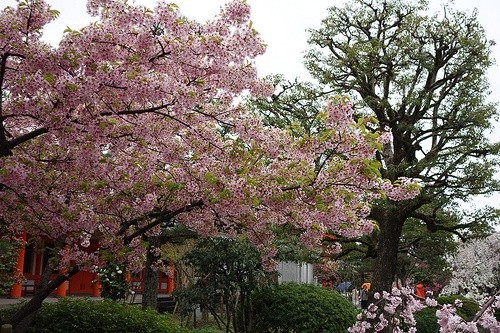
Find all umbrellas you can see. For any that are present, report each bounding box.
[361,283,370,290]
[335,282,352,292]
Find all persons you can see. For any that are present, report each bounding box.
[351,287,359,306]
[416,281,424,301]
[360,284,370,309]
